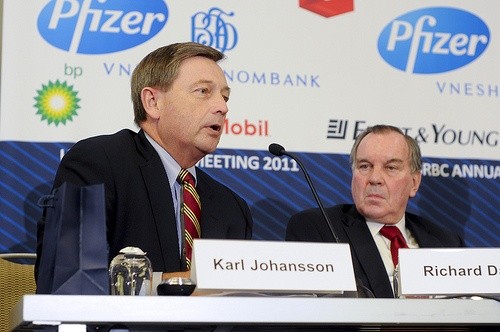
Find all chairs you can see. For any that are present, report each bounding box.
[0,253,39,332]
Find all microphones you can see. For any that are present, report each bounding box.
[269,144,375,299]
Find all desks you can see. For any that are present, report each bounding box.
[9,294,500,332]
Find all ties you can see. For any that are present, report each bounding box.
[379,225,409,269]
[176,169,202,272]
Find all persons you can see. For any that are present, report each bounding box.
[32,40,253,295]
[286,125,464,299]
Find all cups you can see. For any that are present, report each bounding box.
[392,263,403,298]
[109,247,153,295]
